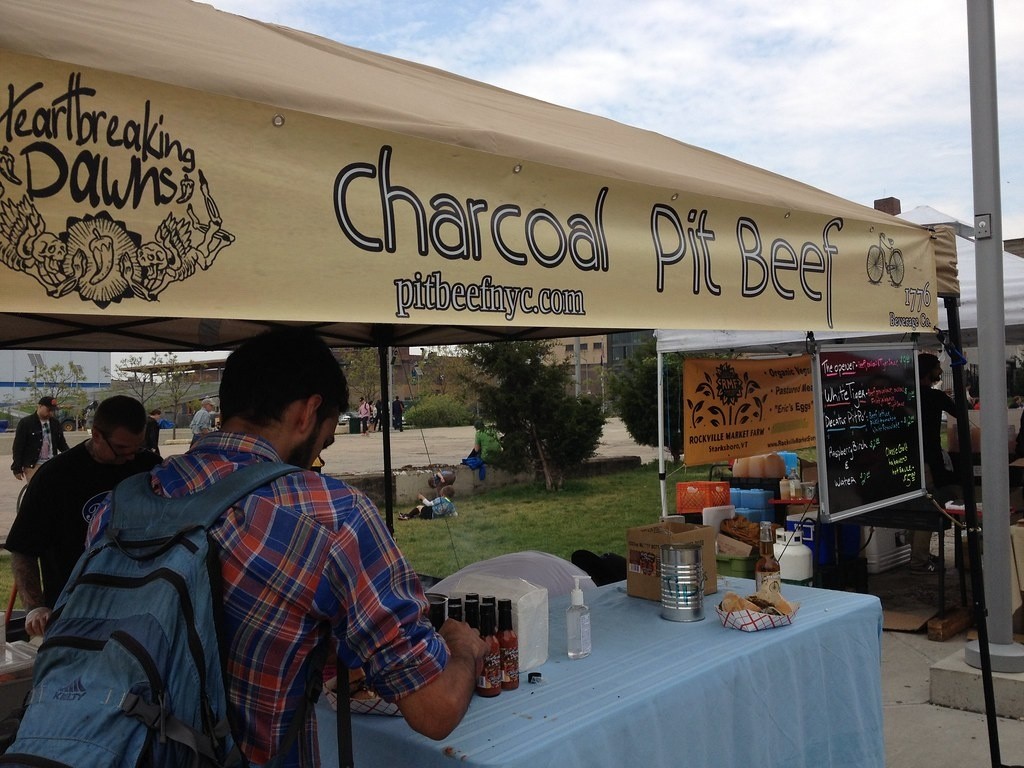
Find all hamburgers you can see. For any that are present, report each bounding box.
[348,668,376,700]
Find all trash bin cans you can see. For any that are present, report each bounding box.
[349,417,361,434]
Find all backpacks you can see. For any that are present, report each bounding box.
[1,458,297,768]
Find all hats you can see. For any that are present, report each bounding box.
[359,397,365,402]
[39,396,61,411]
[201,399,216,406]
[473,420,485,429]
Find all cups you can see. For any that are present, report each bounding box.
[800,482,815,500]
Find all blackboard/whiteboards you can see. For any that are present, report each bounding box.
[811,342,927,524]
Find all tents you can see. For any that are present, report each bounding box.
[0,0,1000,768]
[652,204,1024,621]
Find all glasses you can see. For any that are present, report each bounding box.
[104,440,144,461]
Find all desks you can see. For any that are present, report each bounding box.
[313,575,884,768]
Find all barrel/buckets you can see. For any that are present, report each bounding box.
[658,542,705,622]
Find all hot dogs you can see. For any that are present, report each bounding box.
[721,587,793,614]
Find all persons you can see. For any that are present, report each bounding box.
[4,394,165,635]
[465,420,501,462]
[85,325,489,768]
[905,352,959,575]
[964,381,1021,410]
[356,393,405,436]
[10,394,216,486]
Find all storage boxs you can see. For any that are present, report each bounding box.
[716,555,759,580]
[786,511,860,564]
[626,521,718,601]
[676,481,731,514]
[714,601,801,632]
[721,477,787,500]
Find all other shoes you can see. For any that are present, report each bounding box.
[398,513,410,520]
[911,552,946,574]
[400,429,403,432]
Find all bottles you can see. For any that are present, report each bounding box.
[447,592,521,698]
[0,611,7,664]
[780,467,803,502]
[753,521,781,596]
